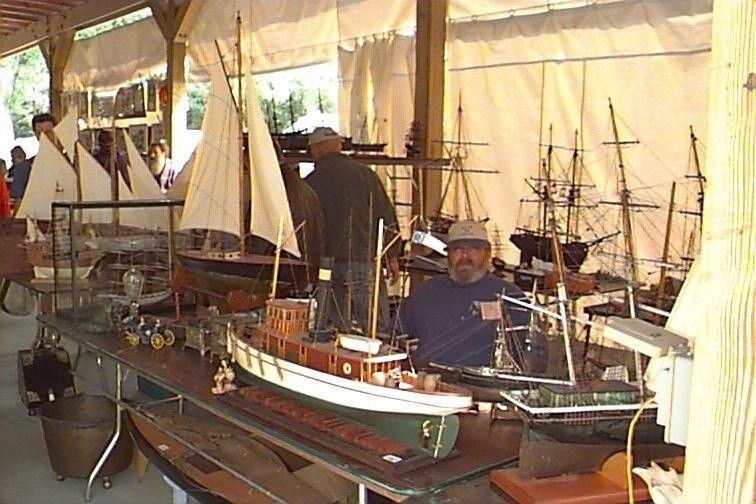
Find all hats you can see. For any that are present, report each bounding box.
[442,220,489,251]
[303,126,342,155]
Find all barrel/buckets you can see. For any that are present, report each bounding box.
[35,393,131,477]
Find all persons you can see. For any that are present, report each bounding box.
[300,125,404,337]
[389,217,551,381]
[0,111,182,221]
[239,134,326,294]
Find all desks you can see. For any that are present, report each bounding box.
[0,215,684,504]
